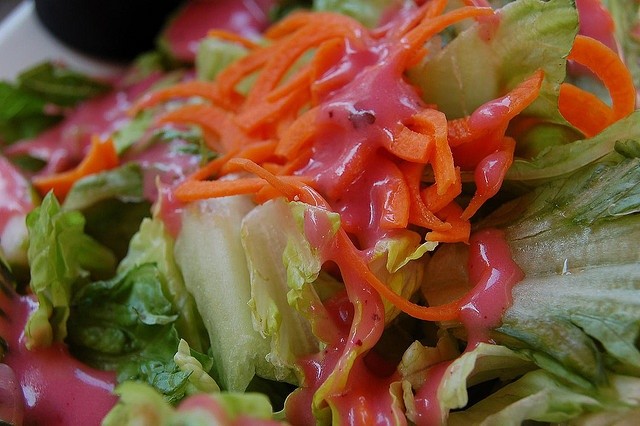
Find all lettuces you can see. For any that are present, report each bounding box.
[407,4,639,425]
[31,186,348,402]
[5,54,113,148]
